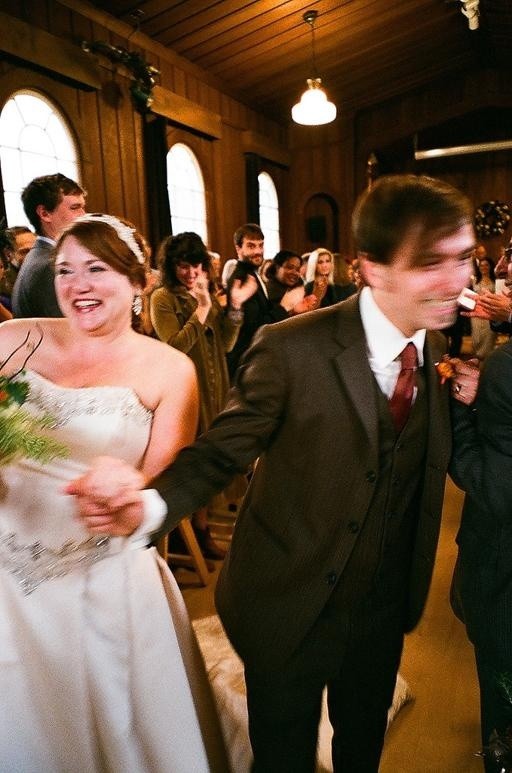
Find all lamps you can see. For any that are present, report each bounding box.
[289,6,339,127]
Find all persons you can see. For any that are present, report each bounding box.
[0,212,202,772]
[446,243,511,360]
[82,175,478,773]
[134,223,363,559]
[0,175,86,321]
[450,236,512,772]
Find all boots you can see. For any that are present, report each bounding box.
[170,525,226,572]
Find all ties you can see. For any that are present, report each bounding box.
[389,342,418,433]
